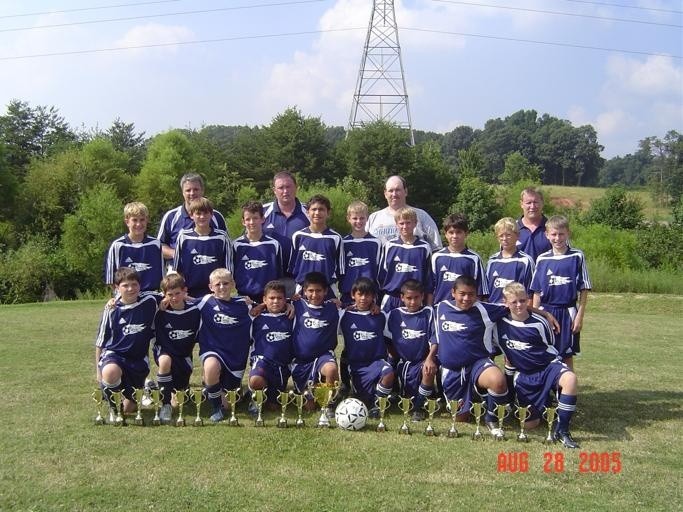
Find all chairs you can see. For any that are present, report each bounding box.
[554,430,578,448]
[143,387,154,405]
[486,421,504,436]
[412,408,425,420]
[325,405,334,418]
[160,404,174,420]
[208,407,226,421]
[372,409,379,417]
[250,402,264,417]
[110,407,123,421]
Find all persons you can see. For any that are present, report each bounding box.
[337,200,388,402]
[158,267,296,423]
[378,207,433,406]
[104,272,253,423]
[287,194,347,298]
[489,281,579,449]
[103,201,163,406]
[290,276,394,420]
[428,212,490,408]
[499,184,570,262]
[530,214,592,430]
[172,196,234,402]
[231,201,284,402]
[364,173,444,255]
[483,216,535,422]
[247,280,342,414]
[157,172,232,405]
[94,267,197,424]
[249,271,382,419]
[430,273,562,437]
[383,278,440,423]
[243,171,331,297]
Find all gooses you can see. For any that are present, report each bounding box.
[90,386,308,429]
[372,392,559,447]
[305,377,341,428]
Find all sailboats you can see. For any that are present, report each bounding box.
[335,398,368,430]
